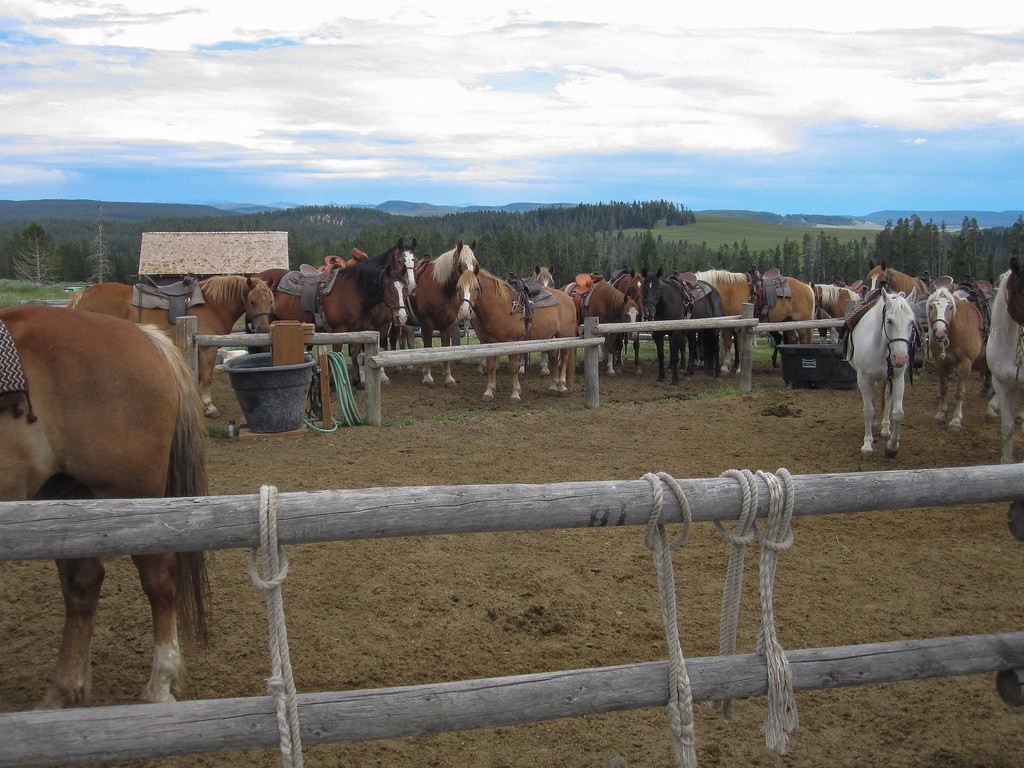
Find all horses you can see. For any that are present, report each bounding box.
[0,235,1023,712]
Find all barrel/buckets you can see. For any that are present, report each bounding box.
[778,344,856,389]
[215,353,317,434]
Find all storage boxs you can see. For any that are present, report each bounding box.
[777,343,858,389]
